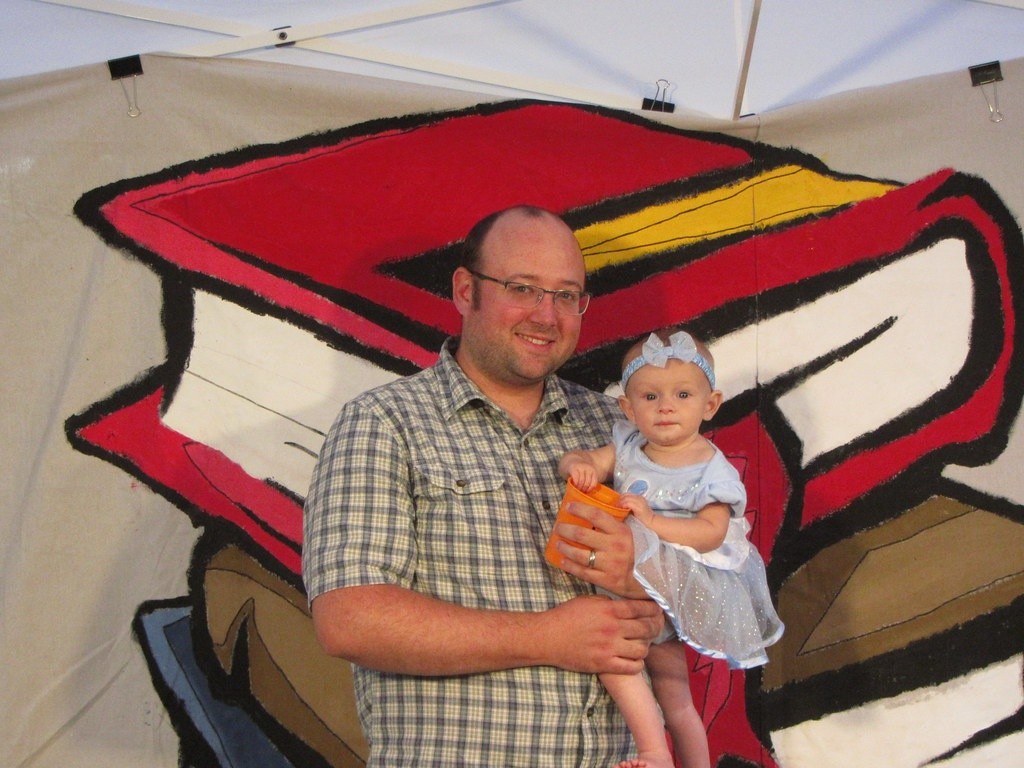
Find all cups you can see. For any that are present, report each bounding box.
[545,477,631,570]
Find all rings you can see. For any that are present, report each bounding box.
[589,550,595,569]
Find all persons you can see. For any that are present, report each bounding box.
[301,205,664,768]
[559,326,786,768]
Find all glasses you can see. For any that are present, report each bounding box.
[465,265,592,316]
